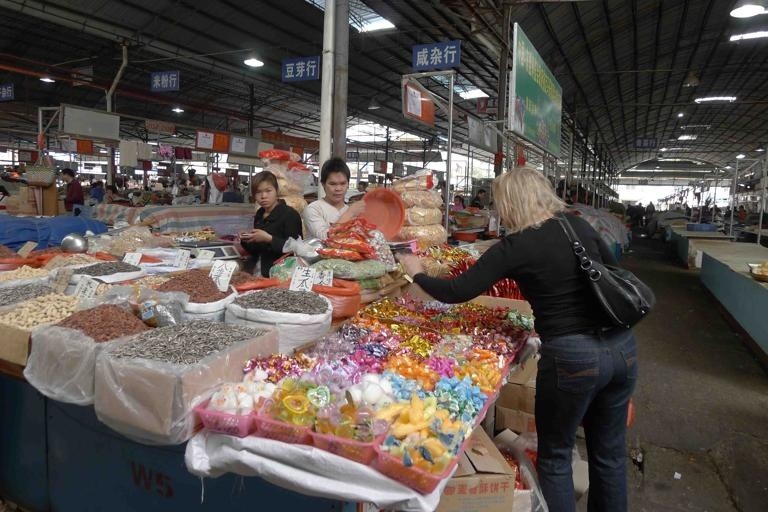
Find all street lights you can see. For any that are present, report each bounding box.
[556,203,660,337]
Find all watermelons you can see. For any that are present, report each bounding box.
[177,240,242,260]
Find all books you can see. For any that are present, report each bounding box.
[30,323,95,408]
[94,326,281,446]
[0,306,32,369]
[494,354,538,434]
[437,429,524,512]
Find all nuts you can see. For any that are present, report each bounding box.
[0,226,328,365]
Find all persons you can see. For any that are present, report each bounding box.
[471,189,486,210]
[626,199,748,232]
[397,165,640,512]
[452,196,466,212]
[6,160,207,220]
[301,157,366,260]
[235,171,303,279]
